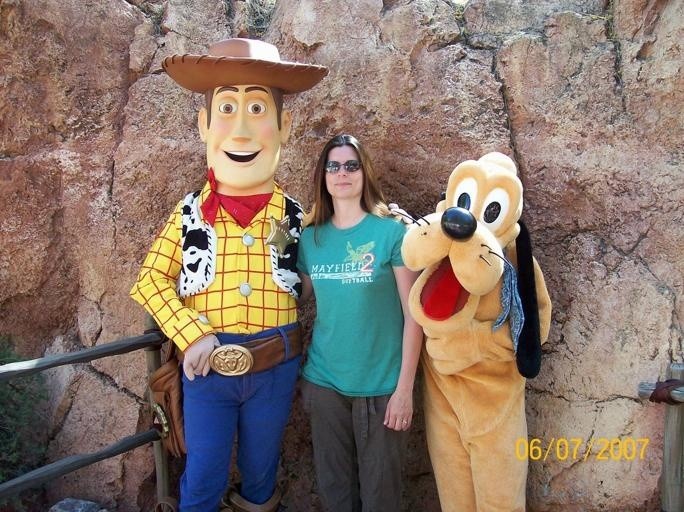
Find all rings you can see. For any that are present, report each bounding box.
[402,420,408,424]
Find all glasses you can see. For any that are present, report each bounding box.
[324,160,361,172]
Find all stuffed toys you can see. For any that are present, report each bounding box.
[389,152,552,512]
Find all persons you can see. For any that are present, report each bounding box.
[128,38,330,512]
[297,134,424,512]
